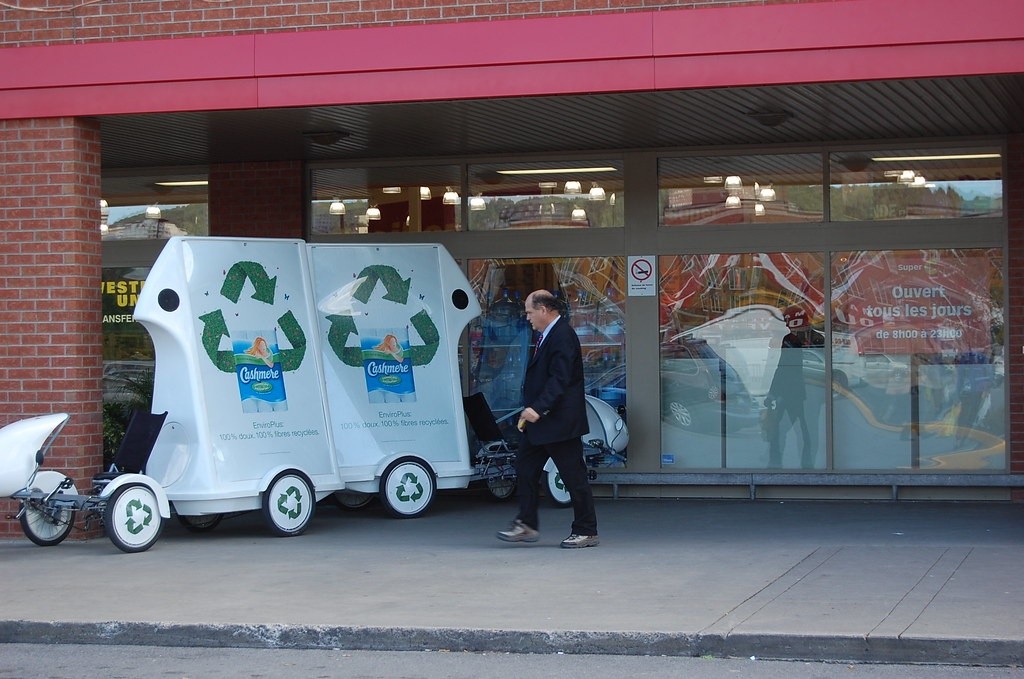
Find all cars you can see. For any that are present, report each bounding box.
[585,331,911,437]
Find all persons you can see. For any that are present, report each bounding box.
[764,305,826,469]
[496,288,600,550]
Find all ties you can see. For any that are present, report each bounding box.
[533,335,543,357]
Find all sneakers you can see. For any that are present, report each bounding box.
[496,519,539,542]
[561,533,599,548]
